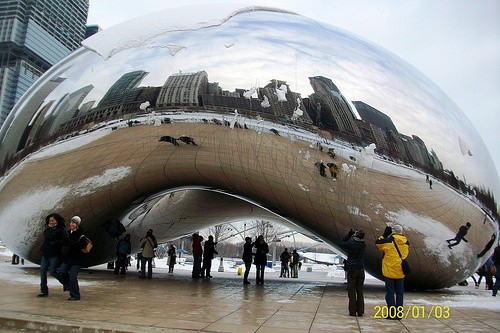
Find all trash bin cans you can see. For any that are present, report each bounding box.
[106,260,114,269]
[266,261,272,268]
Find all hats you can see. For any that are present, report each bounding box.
[70,216,82,225]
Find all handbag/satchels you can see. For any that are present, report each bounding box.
[78,235,93,253]
[402,258,413,274]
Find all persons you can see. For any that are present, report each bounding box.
[474,243,500,295]
[114,233,132,275]
[340,228,364,317]
[241,237,256,285]
[477,233,496,256]
[139,228,158,278]
[200,235,218,277]
[254,234,270,285]
[158,132,199,147]
[59,216,86,299]
[166,244,176,273]
[191,233,203,279]
[110,115,280,136]
[292,250,299,278]
[376,224,410,319]
[36,212,70,297]
[425,174,434,191]
[280,247,289,276]
[310,141,362,176]
[447,220,471,248]
